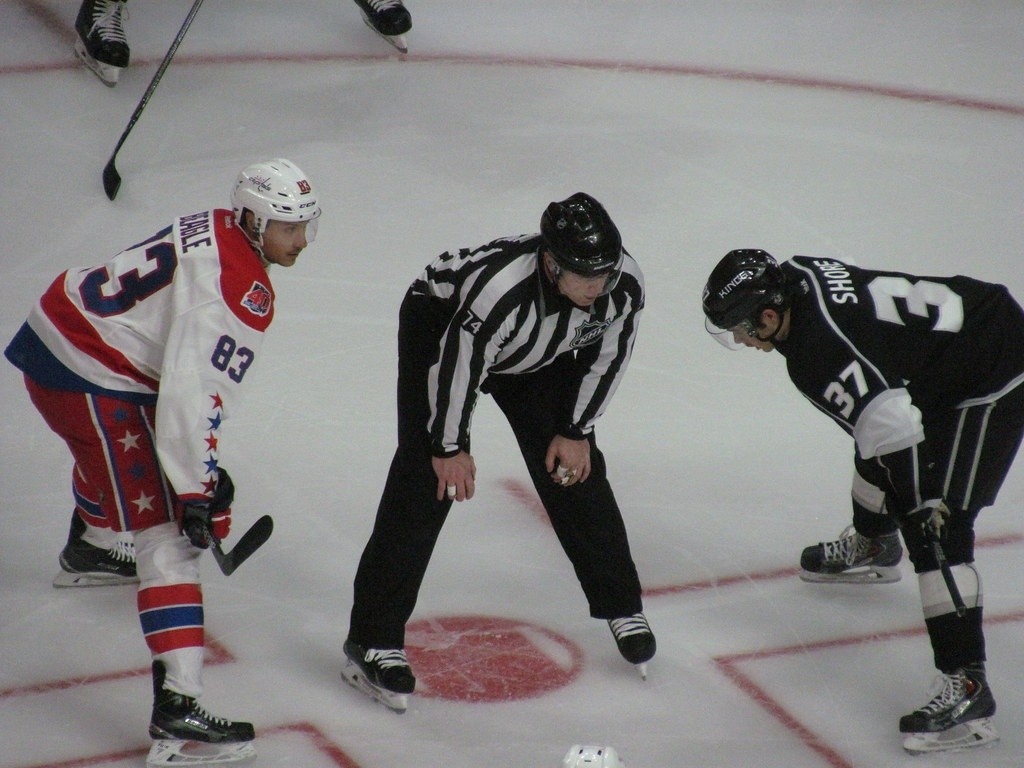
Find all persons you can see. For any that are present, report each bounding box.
[701,249,1024,754]
[342,192,658,713]
[73,0,412,89]
[4,159,321,768]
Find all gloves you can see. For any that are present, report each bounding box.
[176,491,231,550]
[904,495,950,550]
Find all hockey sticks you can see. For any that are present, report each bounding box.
[928,531,969,621]
[203,515,275,577]
[102,1,203,200]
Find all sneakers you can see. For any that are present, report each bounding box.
[800,526,905,585]
[52,510,140,589]
[74,0,130,88]
[340,637,415,716]
[144,659,258,768]
[606,614,656,681]
[899,656,1002,754]
[354,0,413,54]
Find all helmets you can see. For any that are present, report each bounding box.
[541,193,623,277]
[231,158,319,227]
[700,248,785,331]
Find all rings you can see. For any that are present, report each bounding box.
[557,466,568,477]
[447,486,456,497]
